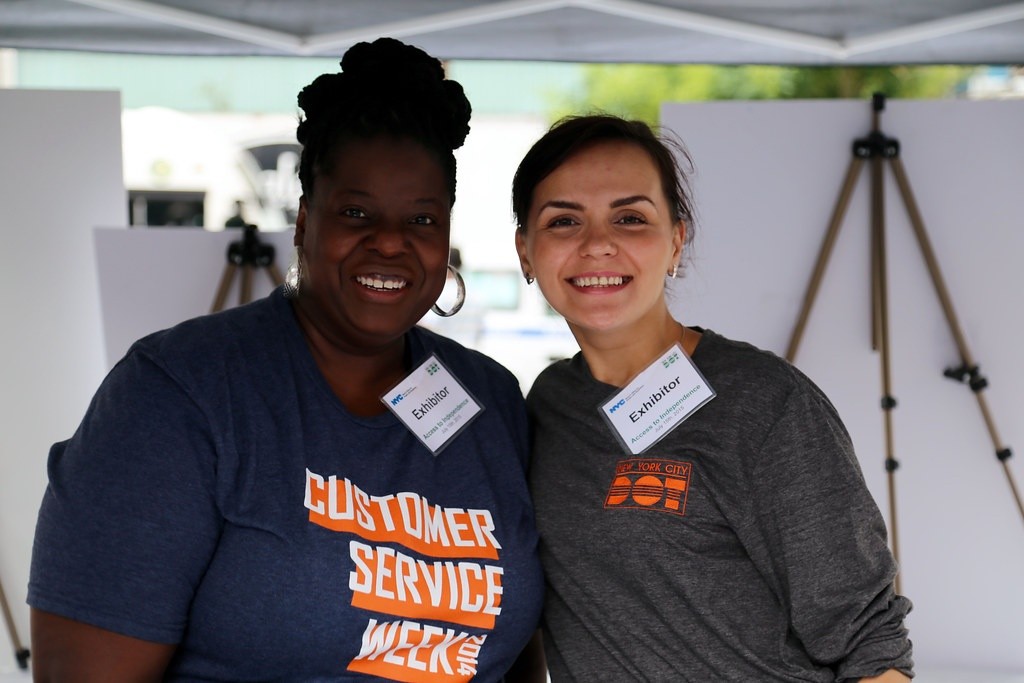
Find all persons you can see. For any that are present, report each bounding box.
[416,247,487,347]
[27,35,546,682]
[250,150,302,230]
[224,201,246,230]
[513,113,917,682]
